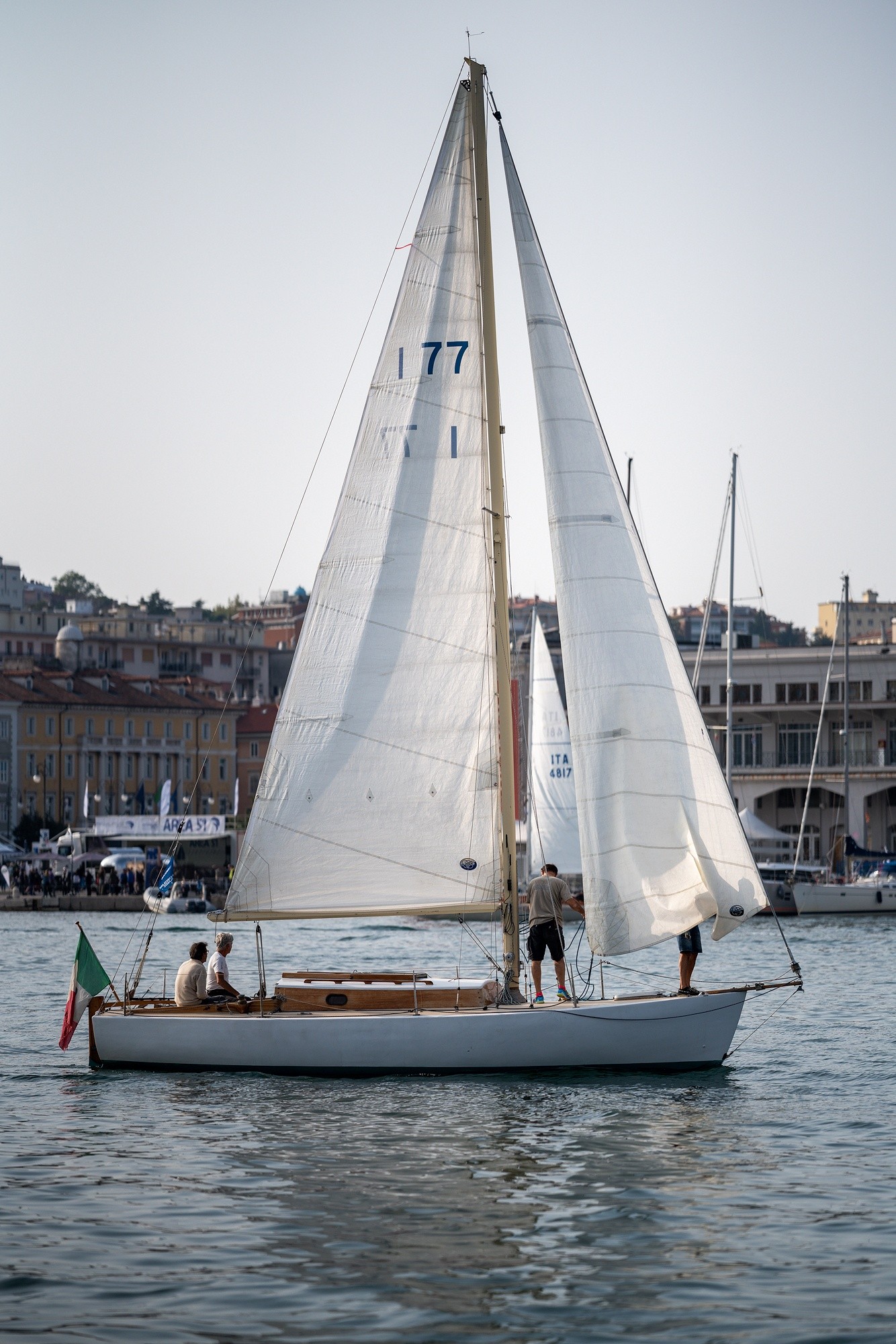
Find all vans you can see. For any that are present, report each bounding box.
[97,853,172,880]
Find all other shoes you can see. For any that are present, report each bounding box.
[678,986,701,995]
[557,988,571,1000]
[532,995,544,1003]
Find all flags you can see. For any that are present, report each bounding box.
[59,929,111,1054]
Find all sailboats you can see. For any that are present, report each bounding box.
[791,573,896,917]
[84,30,806,1080]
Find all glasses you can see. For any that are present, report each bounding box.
[206,950,209,954]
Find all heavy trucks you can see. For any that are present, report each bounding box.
[53,830,110,891]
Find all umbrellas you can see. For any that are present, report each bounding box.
[18,852,36,860]
[30,851,67,861]
[70,851,106,863]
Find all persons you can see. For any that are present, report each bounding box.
[0,861,235,899]
[525,864,585,1004]
[677,925,704,996]
[206,933,251,1001]
[174,942,226,1007]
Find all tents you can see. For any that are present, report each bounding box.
[0,843,16,860]
[738,807,798,862]
[101,853,176,869]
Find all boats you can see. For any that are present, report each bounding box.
[755,861,827,916]
[142,880,217,915]
